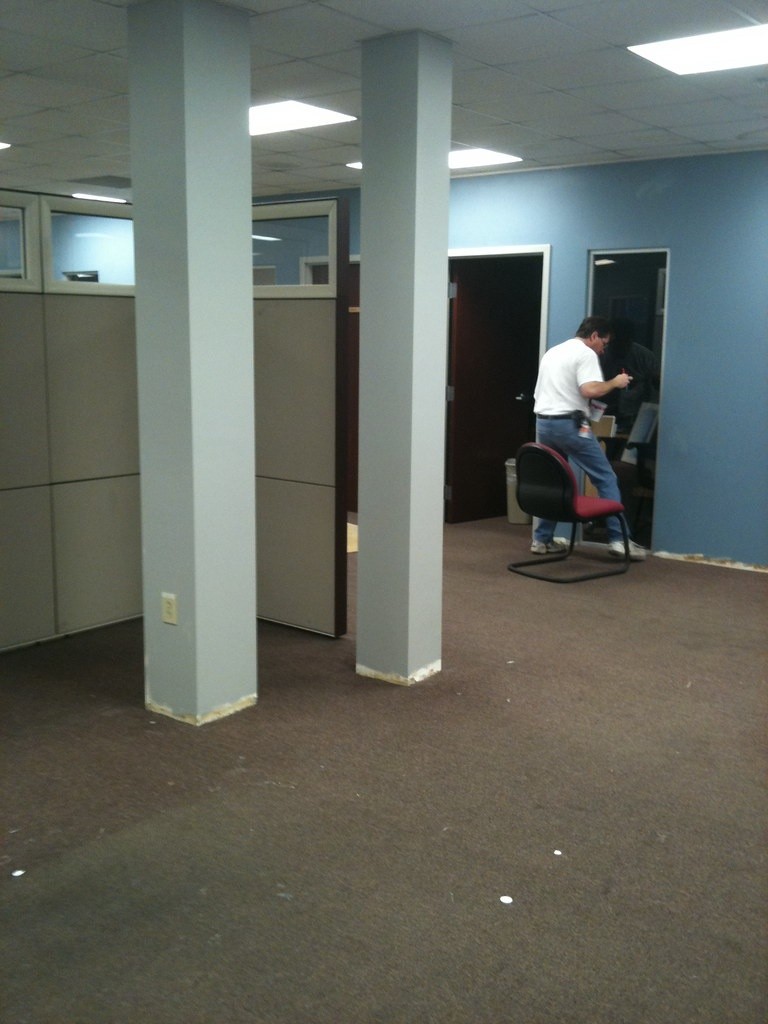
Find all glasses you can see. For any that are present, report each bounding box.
[591,329,608,350]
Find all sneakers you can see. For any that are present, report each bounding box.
[531,536,567,553]
[608,539,647,561]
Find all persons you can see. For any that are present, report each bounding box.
[600,319,659,462]
[531,314,650,560]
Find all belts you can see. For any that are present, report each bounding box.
[537,414,574,420]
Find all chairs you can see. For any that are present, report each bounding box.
[597,436,654,542]
[507,443,630,585]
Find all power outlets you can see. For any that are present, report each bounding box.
[160,593,176,624]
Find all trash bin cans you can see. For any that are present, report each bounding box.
[505,458,532,525]
